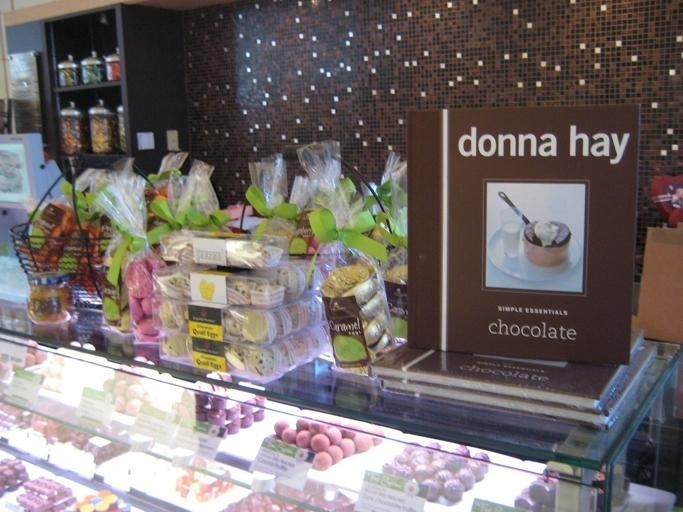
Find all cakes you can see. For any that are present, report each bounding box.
[523,221,571,267]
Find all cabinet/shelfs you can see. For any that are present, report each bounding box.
[0,290,683,512]
[41,0,193,184]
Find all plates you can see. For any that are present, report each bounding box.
[487,216,580,281]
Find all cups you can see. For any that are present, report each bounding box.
[498,208,523,262]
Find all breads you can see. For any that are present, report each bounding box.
[149,260,328,384]
[124,250,172,348]
[157,231,283,271]
[21,181,233,336]
[318,259,393,352]
[249,186,394,333]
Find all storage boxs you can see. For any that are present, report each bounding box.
[636,221,683,343]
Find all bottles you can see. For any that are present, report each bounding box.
[57,48,126,153]
[26,271,74,325]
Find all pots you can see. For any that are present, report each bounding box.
[498,192,571,266]
